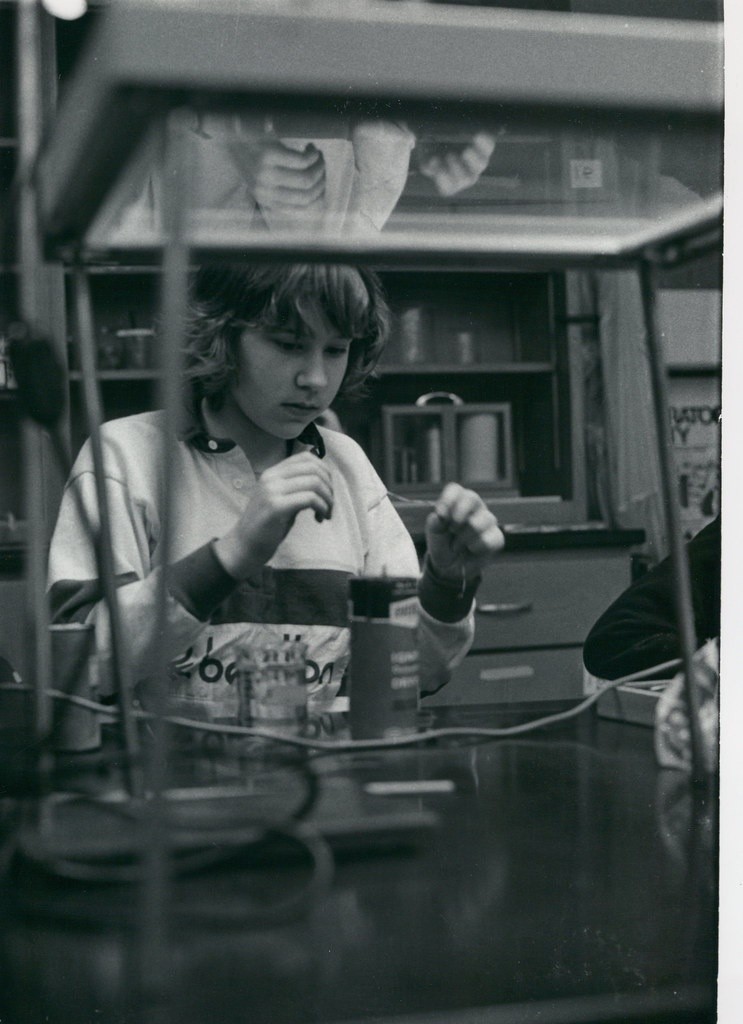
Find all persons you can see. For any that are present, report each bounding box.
[84,117,496,245]
[583,512,721,679]
[46,257,507,717]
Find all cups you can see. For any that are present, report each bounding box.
[48,622,102,753]
[233,642,310,739]
